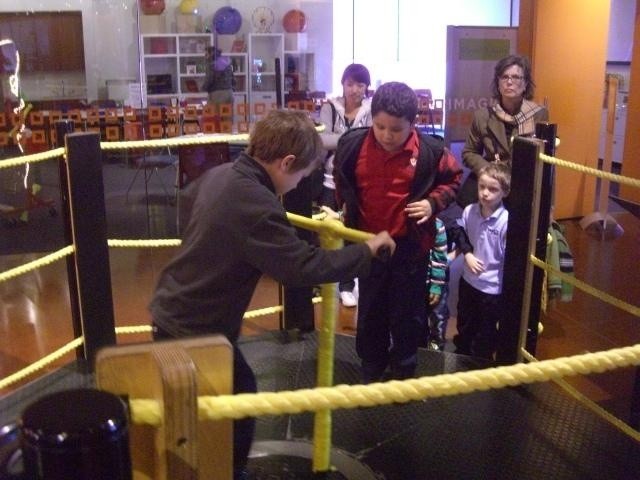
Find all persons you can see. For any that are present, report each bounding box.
[458,54,554,363]
[0,42,27,199]
[426,132,573,363]
[145,107,397,480]
[200,45,237,127]
[331,80,464,385]
[317,62,374,309]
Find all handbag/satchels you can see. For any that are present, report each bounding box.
[307,103,338,204]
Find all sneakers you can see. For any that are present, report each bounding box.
[393,365,416,380]
[361,348,390,378]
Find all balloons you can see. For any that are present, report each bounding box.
[282,9,309,34]
[212,7,243,36]
[178,0,200,15]
[139,0,166,16]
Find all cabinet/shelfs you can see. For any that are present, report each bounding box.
[141,32,214,112]
[246,32,284,122]
[288,51,315,94]
[216,52,248,130]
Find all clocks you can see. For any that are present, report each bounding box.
[252,6,275,33]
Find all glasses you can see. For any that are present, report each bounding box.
[502,74,523,83]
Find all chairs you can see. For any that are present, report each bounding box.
[125,120,176,208]
[176,142,232,189]
